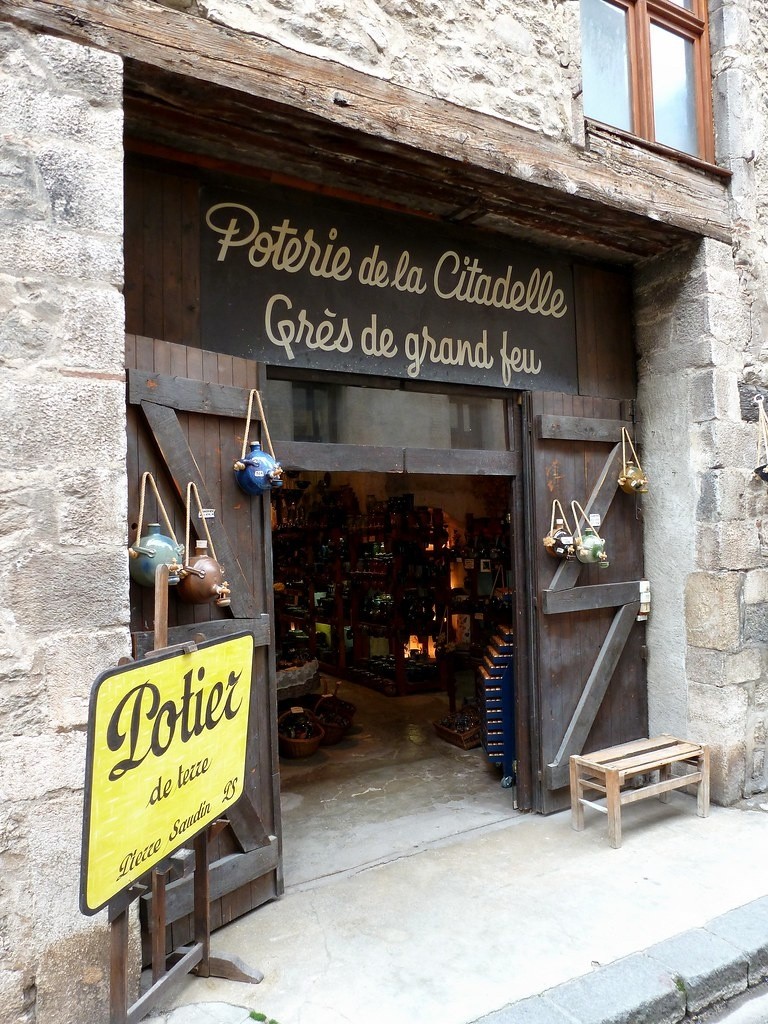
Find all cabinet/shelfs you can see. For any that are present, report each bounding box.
[270,482,516,787]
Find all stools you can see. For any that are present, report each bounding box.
[570,733,711,849]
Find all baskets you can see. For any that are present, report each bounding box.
[278,708,325,758]
[314,696,349,746]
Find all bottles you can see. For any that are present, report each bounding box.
[576,527,605,563]
[617,461,648,495]
[233,440,283,496]
[543,518,575,559]
[176,539,226,605]
[128,522,184,585]
